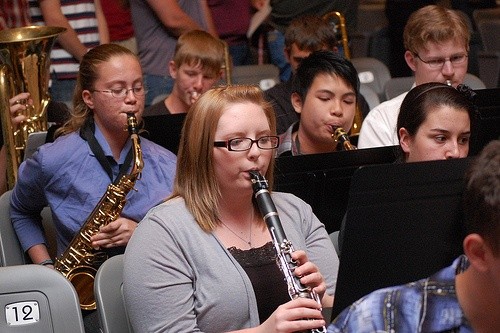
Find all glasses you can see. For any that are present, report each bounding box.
[214,136,280,152]
[94,84,149,98]
[415,51,471,68]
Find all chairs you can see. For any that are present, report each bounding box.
[0,183,133,333]
[386,67,486,100]
[352,57,391,93]
[356,79,380,116]
[230,64,280,88]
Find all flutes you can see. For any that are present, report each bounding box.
[247,169,327,333]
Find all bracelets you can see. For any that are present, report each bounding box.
[39,260,54,265]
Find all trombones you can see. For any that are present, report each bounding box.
[189,41,232,98]
[321,10,364,138]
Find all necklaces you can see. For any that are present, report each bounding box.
[220,202,257,249]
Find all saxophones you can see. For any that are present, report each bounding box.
[53,113,145,309]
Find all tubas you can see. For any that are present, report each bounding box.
[0,26,68,191]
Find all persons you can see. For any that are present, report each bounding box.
[0,0,484,163]
[10,43,178,269]
[122,85,339,333]
[325,139,500,333]
